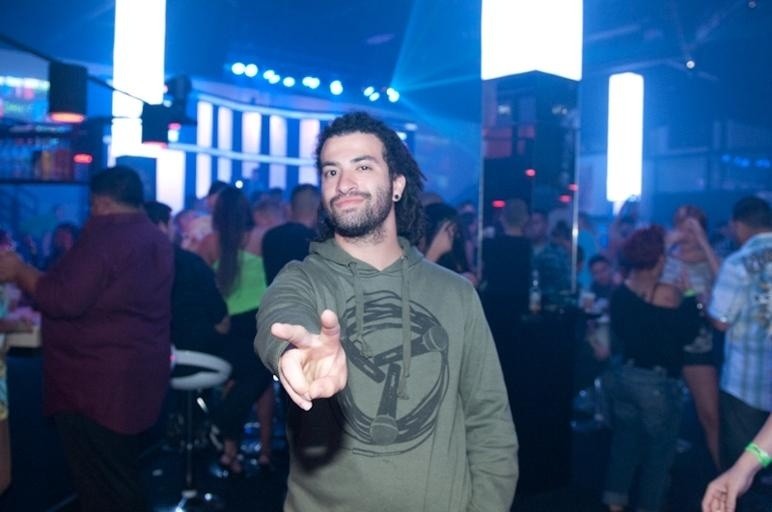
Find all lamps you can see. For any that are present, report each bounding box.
[0,32,193,164]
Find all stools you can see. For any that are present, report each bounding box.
[168,343,233,512]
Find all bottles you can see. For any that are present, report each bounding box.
[528,270,542,321]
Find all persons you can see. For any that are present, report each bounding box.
[528,208,618,421]
[0,165,174,512]
[148,183,334,480]
[254,112,520,511]
[600,195,772,512]
[415,196,534,341]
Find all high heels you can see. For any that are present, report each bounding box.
[218,455,275,479]
